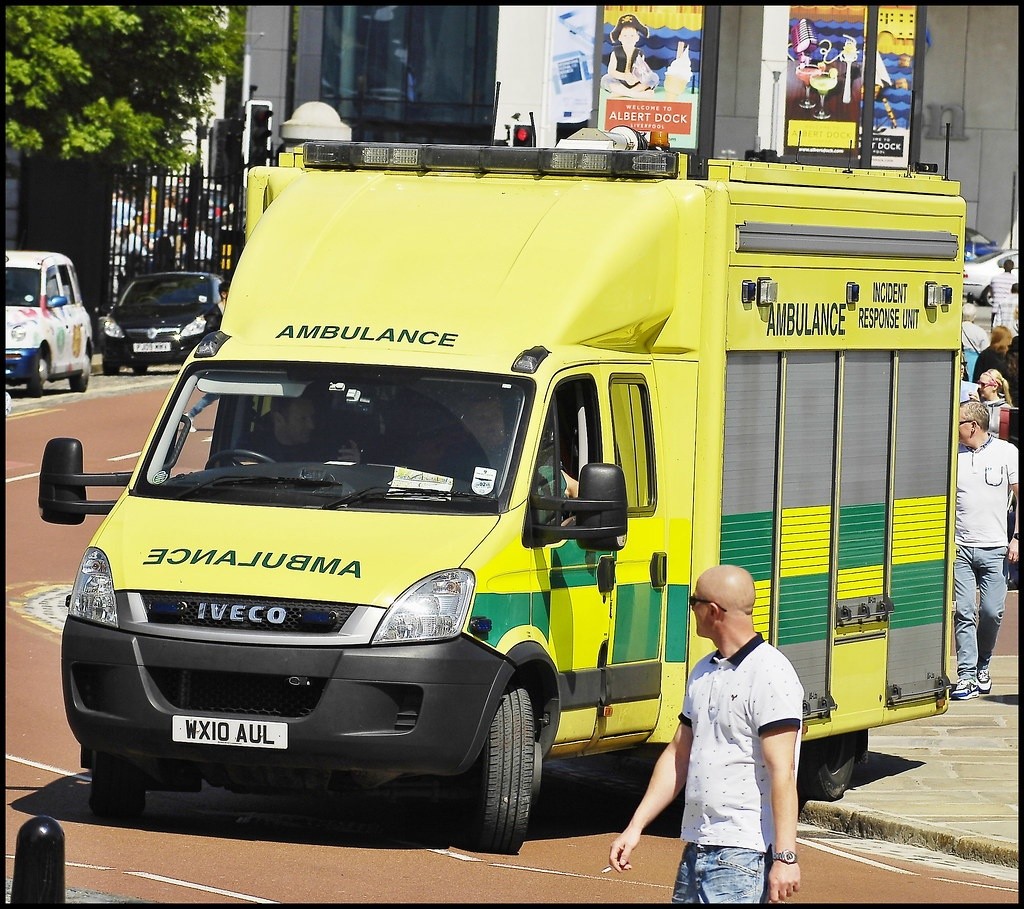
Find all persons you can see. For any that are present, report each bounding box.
[231,392,360,465]
[202,280,231,338]
[608,565,804,903]
[959,281,1019,440]
[435,397,580,529]
[950,398,1018,700]
[602,11,659,98]
[990,259,1018,331]
[113,187,213,272]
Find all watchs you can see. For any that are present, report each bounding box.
[1014,533,1019,540]
[773,849,797,864]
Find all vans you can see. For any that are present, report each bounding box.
[5,250,94,399]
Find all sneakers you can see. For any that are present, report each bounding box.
[977,670,992,693]
[950,679,980,700]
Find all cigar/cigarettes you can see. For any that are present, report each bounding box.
[601,862,620,873]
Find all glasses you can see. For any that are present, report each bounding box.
[978,380,995,387]
[688,596,728,612]
[961,362,967,366]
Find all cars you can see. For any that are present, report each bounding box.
[963,248,1019,308]
[99,270,224,379]
[963,227,1000,261]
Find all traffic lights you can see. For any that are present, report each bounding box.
[210,119,242,179]
[513,124,533,148]
[241,100,273,187]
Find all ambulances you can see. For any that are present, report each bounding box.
[40,127,967,857]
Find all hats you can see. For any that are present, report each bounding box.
[610,14,650,42]
